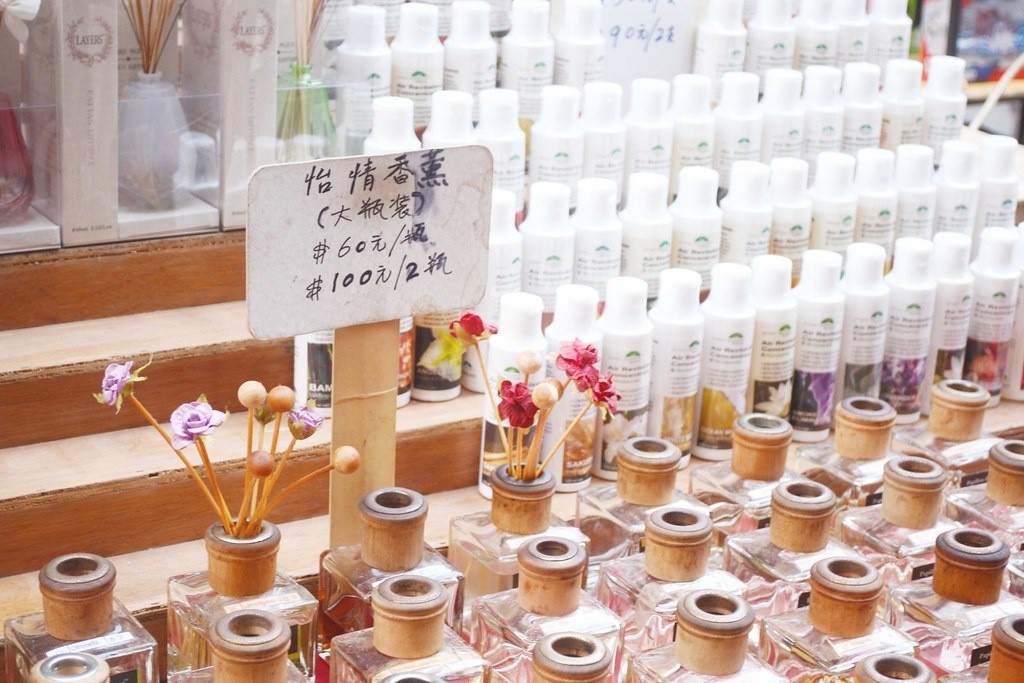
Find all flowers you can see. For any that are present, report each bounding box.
[416,312,622,483]
[0,0,43,56]
[93,354,361,541]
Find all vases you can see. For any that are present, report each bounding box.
[119,71,194,210]
[0,90,37,225]
[275,61,338,166]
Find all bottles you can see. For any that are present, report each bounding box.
[1,373,1024,683]
[293,0,1024,498]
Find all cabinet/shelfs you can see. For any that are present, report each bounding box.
[0,230,618,683]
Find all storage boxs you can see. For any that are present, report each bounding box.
[25,0,119,249]
[178,1,282,229]
